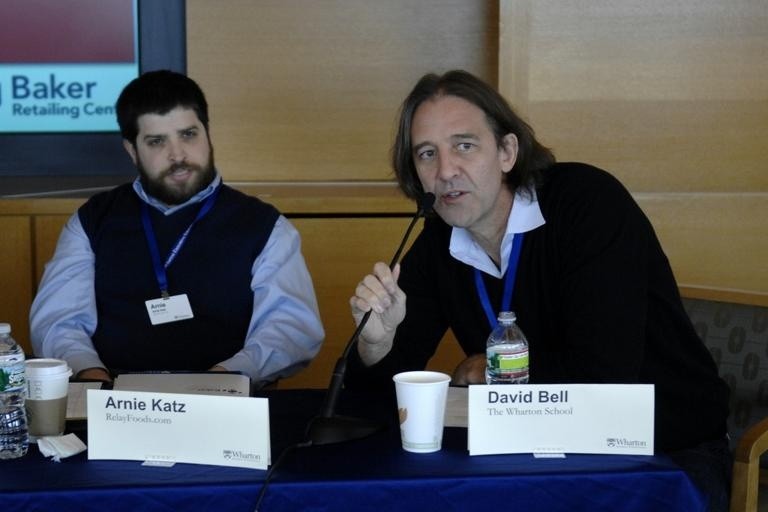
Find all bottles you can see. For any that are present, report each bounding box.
[0,322,30,460]
[485,310,530,384]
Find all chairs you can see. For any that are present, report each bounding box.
[677,285,768,512]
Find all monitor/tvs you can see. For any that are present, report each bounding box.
[0,0,189,200]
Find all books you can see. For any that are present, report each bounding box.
[113,368,253,396]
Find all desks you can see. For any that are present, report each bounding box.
[0,391,711,512]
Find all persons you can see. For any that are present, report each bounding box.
[340,67,737,512]
[29,68,327,388]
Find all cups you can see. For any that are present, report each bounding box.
[25,358,74,444]
[392,370,452,454]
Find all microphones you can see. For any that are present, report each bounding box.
[306,190,437,465]
